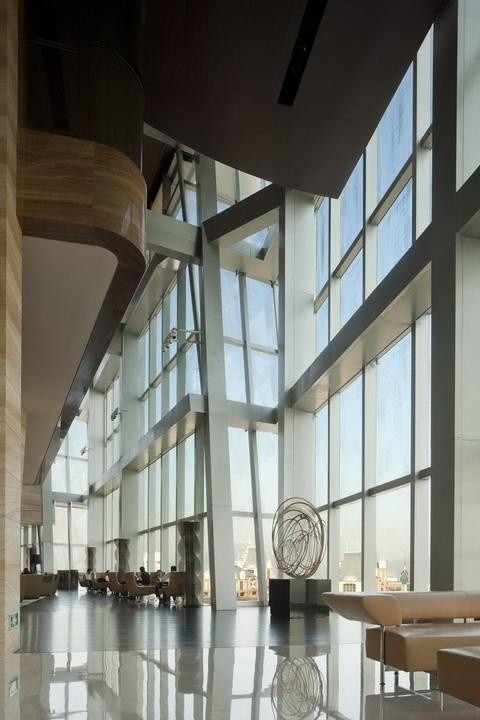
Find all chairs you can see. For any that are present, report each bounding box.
[20,574,59,603]
[78,572,185,607]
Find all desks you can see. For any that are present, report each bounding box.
[437,646,480,711]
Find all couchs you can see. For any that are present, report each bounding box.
[324,591,480,689]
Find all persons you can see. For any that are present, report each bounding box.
[21,568,31,574]
[86,568,92,588]
[104,570,112,591]
[157,565,177,603]
[154,569,166,597]
[139,567,150,585]
